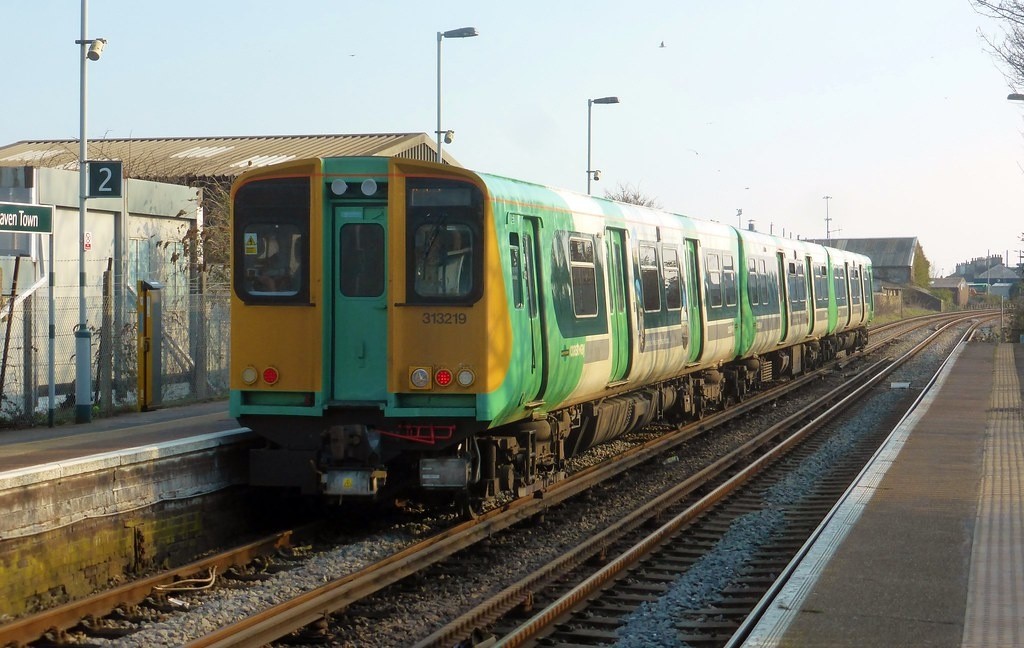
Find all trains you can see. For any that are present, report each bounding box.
[227,156,874,521]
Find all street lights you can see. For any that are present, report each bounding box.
[438,27,479,164]
[588,97,620,195]
